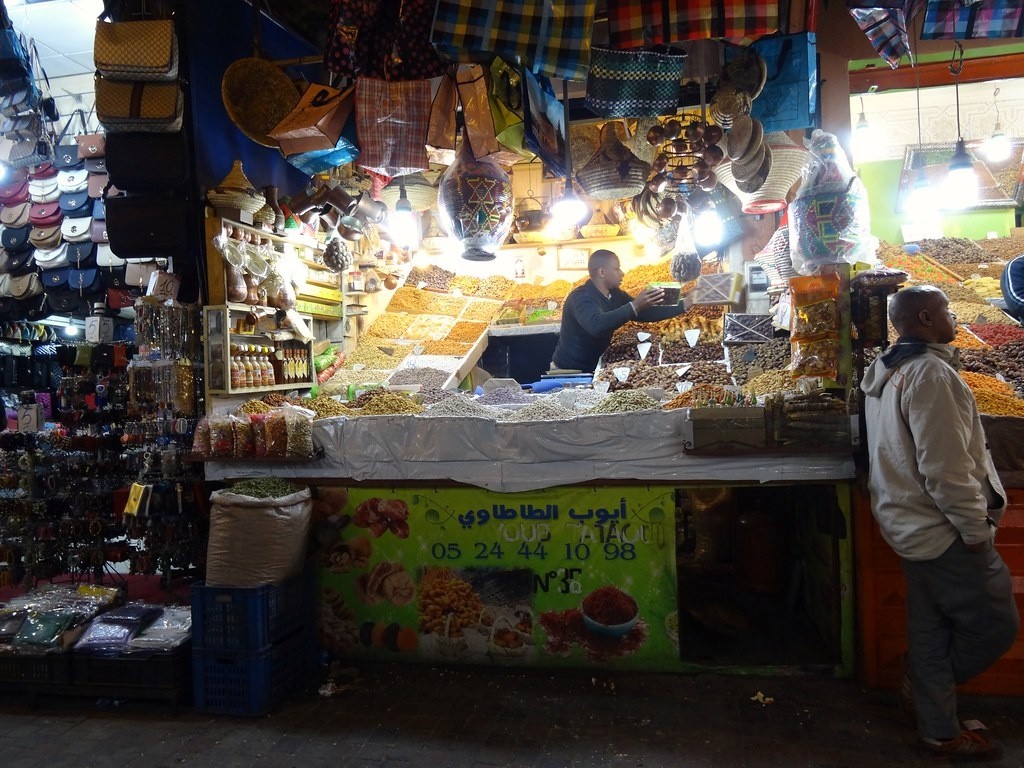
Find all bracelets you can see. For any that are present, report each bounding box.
[0,431,106,584]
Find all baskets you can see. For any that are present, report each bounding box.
[0,655,190,689]
[584,41,688,120]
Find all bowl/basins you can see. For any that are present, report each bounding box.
[580,591,639,638]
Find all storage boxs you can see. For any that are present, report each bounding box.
[71,649,189,688]
[0,652,71,693]
[188,576,315,717]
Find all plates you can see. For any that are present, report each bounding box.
[665,611,678,642]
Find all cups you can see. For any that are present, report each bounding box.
[246,305,268,325]
[275,310,291,329]
[288,186,382,228]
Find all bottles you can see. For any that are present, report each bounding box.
[720,389,744,408]
[709,390,717,409]
[751,393,757,407]
[690,389,708,410]
[210,343,310,389]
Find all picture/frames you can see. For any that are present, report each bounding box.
[557,248,593,271]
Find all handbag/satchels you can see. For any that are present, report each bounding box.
[268,56,569,176]
[325,0,447,82]
[430,0,594,83]
[749,31,817,133]
[848,0,926,70]
[0,6,186,310]
[920,0,1024,40]
[606,0,782,49]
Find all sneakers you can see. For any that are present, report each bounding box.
[917,731,1004,762]
[898,654,915,714]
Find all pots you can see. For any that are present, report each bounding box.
[338,215,365,241]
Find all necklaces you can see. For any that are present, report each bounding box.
[124,305,203,484]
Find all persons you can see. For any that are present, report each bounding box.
[1000,254,1024,324]
[859,285,1019,758]
[550,249,701,373]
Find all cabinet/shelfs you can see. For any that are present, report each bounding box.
[198,206,368,417]
